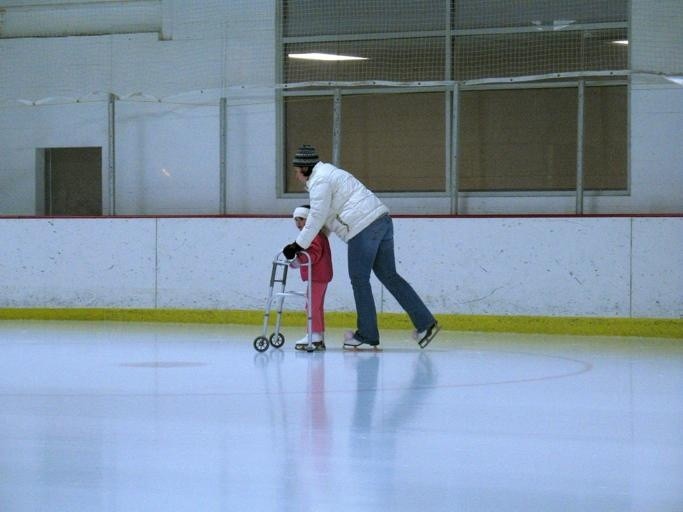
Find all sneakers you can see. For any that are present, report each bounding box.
[344,338,377,346]
[418,321,436,344]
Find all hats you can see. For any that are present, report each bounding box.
[293,205,310,219]
[292,144,319,167]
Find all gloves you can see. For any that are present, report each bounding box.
[283,242,304,259]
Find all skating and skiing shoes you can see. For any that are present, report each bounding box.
[295,334,325,351]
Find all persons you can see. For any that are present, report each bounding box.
[290,205,333,344]
[293,144,437,346]
